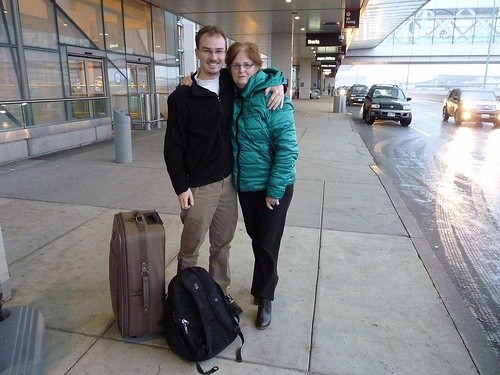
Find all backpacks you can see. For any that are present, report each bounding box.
[162,266,242,363]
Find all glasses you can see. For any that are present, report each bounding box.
[230,63,255,69]
[198,48,227,55]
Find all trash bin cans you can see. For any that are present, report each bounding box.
[333,96,346,113]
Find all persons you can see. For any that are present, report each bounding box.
[180,42,299,330]
[327,83,331,96]
[163,24,288,314]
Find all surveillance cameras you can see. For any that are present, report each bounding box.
[338,35,344,42]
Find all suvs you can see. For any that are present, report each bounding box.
[346,84,370,107]
[363,84,413,126]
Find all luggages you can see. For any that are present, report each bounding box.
[108,209,165,338]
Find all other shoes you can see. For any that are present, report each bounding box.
[226,294,243,314]
[253,295,272,330]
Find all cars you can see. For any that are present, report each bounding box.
[442,87,500,128]
[334,85,351,96]
